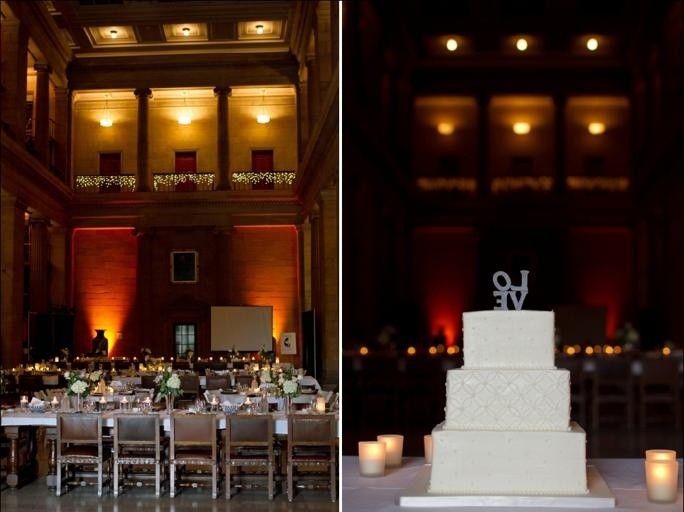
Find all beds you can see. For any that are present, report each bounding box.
[341,453,684,512]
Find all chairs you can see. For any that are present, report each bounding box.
[342,342,684,435]
[0,353,341,506]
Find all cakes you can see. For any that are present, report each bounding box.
[428,269,589,496]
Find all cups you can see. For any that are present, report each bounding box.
[19,392,326,415]
[357,440,387,479]
[376,433,408,469]
[422,434,432,466]
[642,448,678,504]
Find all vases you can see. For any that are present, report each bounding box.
[90,328,109,358]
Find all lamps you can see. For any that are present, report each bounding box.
[254,25,263,35]
[255,89,271,125]
[98,94,113,128]
[176,91,192,126]
[181,27,191,36]
[109,30,118,39]
